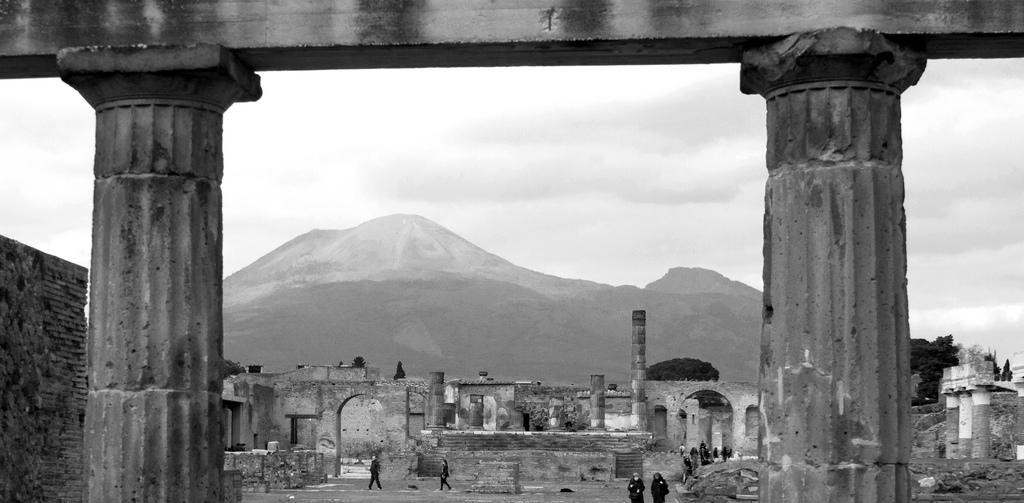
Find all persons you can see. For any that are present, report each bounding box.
[650,471,669,503]
[368,454,382,491]
[628,472,646,503]
[439,458,451,491]
[679,439,733,476]
